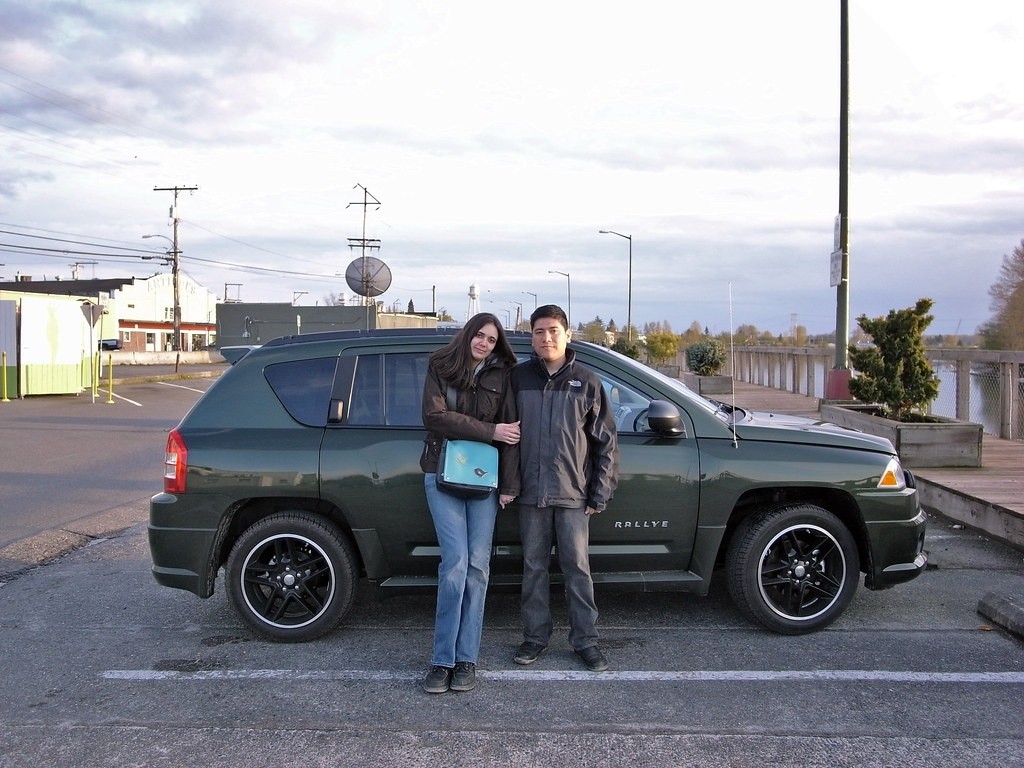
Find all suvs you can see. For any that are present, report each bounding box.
[145,328,936,644]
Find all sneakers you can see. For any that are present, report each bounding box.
[574,646,609,671]
[424,666,452,692]
[450,661,476,690]
[514,641,548,664]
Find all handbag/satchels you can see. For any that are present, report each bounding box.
[436,438,499,499]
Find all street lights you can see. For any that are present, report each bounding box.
[548,270,571,330]
[522,291,537,308]
[509,300,523,326]
[599,230,632,342]
[501,314,508,328]
[142,234,181,351]
[505,309,511,328]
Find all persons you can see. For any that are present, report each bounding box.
[420,304,617,694]
